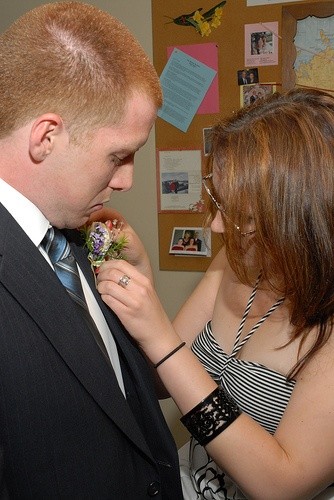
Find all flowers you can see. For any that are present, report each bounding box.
[160,0,229,38]
[83,216,130,273]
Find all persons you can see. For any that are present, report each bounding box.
[238,72,263,106]
[0,0,188,500]
[172,231,203,251]
[253,36,272,55]
[85,83,334,499]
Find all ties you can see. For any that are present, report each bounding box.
[42,228,124,401]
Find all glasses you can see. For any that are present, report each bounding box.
[199,172,260,257]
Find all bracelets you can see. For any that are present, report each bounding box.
[152,341,186,369]
[180,389,240,445]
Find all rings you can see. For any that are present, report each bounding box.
[118,275,130,287]
